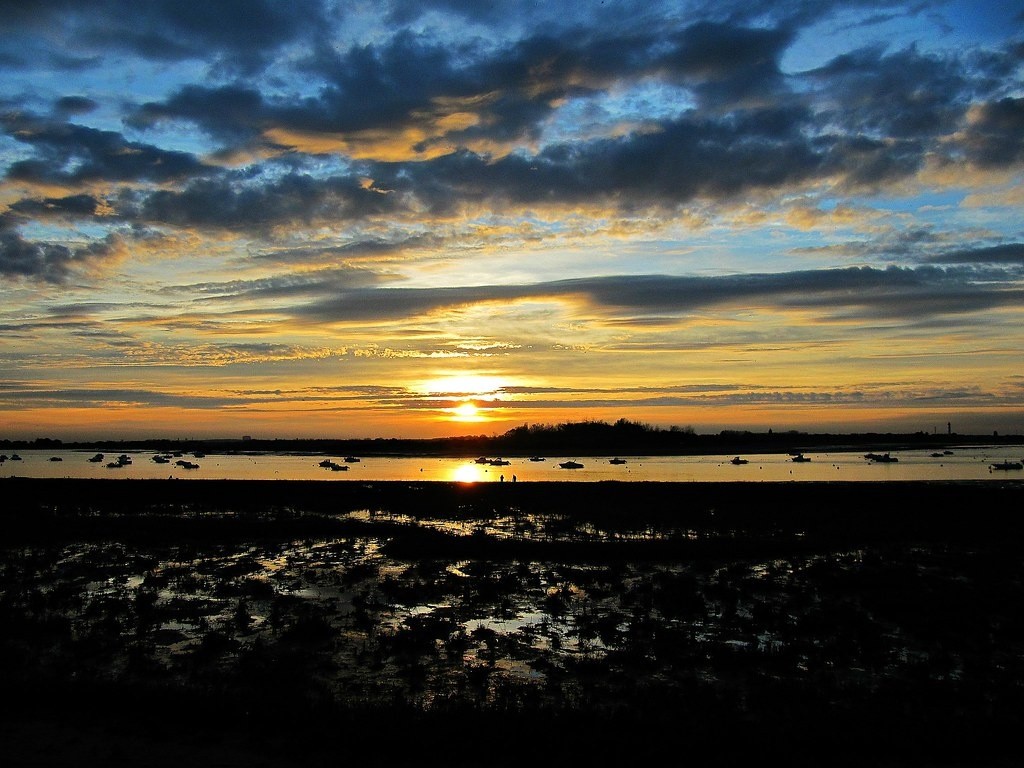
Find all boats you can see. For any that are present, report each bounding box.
[792,453,811,464]
[731,455,750,464]
[862,451,901,463]
[87,451,132,468]
[609,458,625,464]
[151,449,206,470]
[931,453,945,458]
[343,454,359,462]
[473,457,511,465]
[991,459,1024,471]
[559,460,585,469]
[529,455,546,462]
[319,459,348,471]
